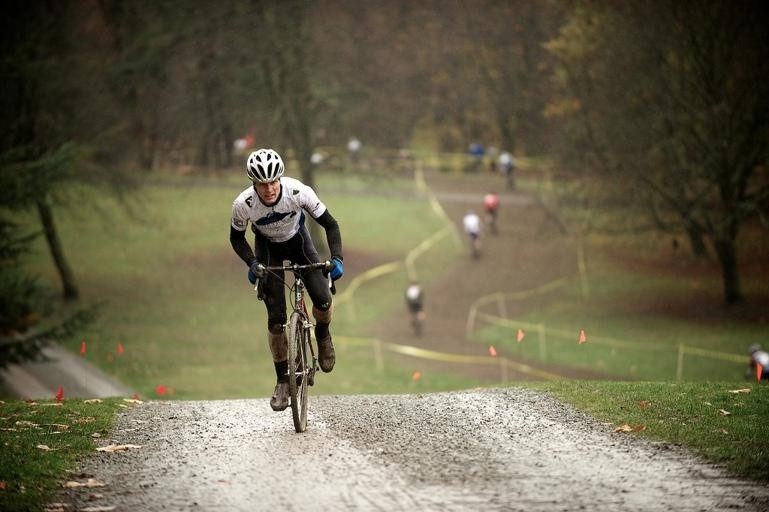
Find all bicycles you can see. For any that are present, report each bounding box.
[253,260,335,434]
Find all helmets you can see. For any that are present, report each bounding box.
[247,148,285,183]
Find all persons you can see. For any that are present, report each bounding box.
[497,150,517,172]
[309,150,333,166]
[229,148,343,411]
[745,343,769,385]
[347,135,362,163]
[404,279,423,315]
[468,139,483,157]
[482,191,500,217]
[461,209,481,237]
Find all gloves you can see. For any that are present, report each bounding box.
[323,258,343,281]
[248,263,265,283]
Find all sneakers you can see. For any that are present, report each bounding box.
[315,326,335,372]
[270,382,290,411]
